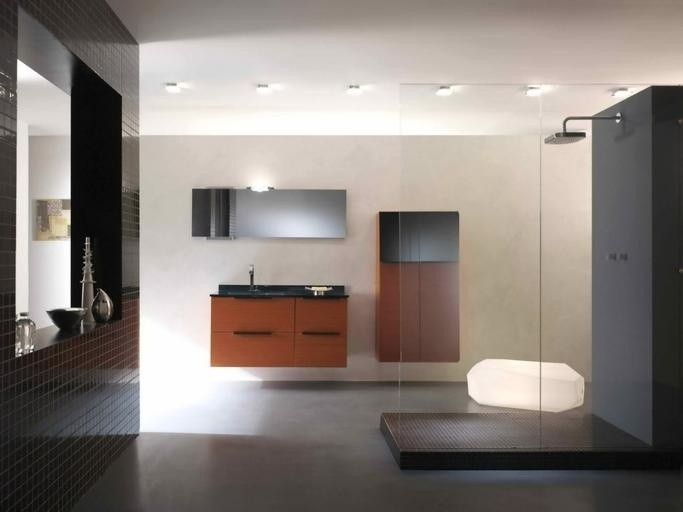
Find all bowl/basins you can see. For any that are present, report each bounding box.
[46,308,87,333]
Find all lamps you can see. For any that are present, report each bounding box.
[152,80,653,97]
[466,357,586,414]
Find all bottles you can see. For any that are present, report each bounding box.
[15,311,36,355]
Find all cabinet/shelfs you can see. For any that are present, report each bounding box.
[376,263,460,363]
[210,297,295,367]
[295,298,347,367]
[191,188,229,237]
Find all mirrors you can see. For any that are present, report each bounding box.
[230,189,347,239]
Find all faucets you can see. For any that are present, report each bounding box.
[249,264,254,292]
